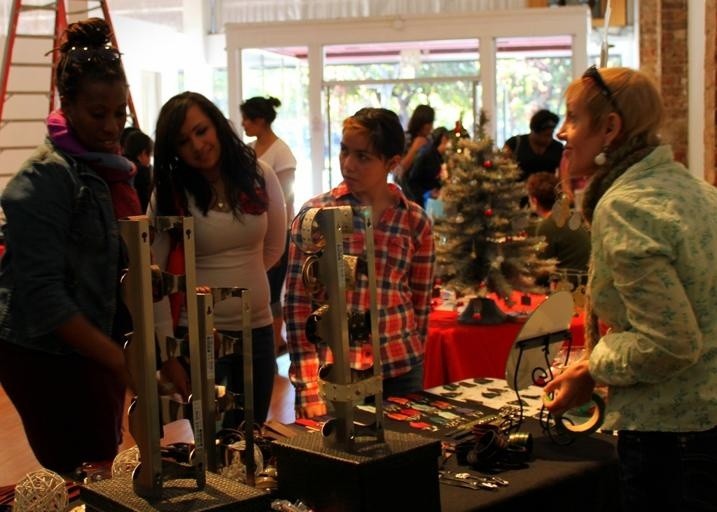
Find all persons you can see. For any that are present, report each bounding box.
[239,94,299,357]
[281,106,437,419]
[393,102,434,184]
[401,128,450,208]
[526,170,591,286]
[123,132,156,211]
[146,88,289,468]
[559,148,588,202]
[500,110,562,211]
[1,18,138,483]
[540,64,715,511]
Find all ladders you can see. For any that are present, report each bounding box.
[0,1,139,177]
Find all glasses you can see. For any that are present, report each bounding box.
[581,64,623,120]
[61,44,122,79]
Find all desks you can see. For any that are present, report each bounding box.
[419,289,605,388]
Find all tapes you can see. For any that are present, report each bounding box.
[550,392,604,439]
